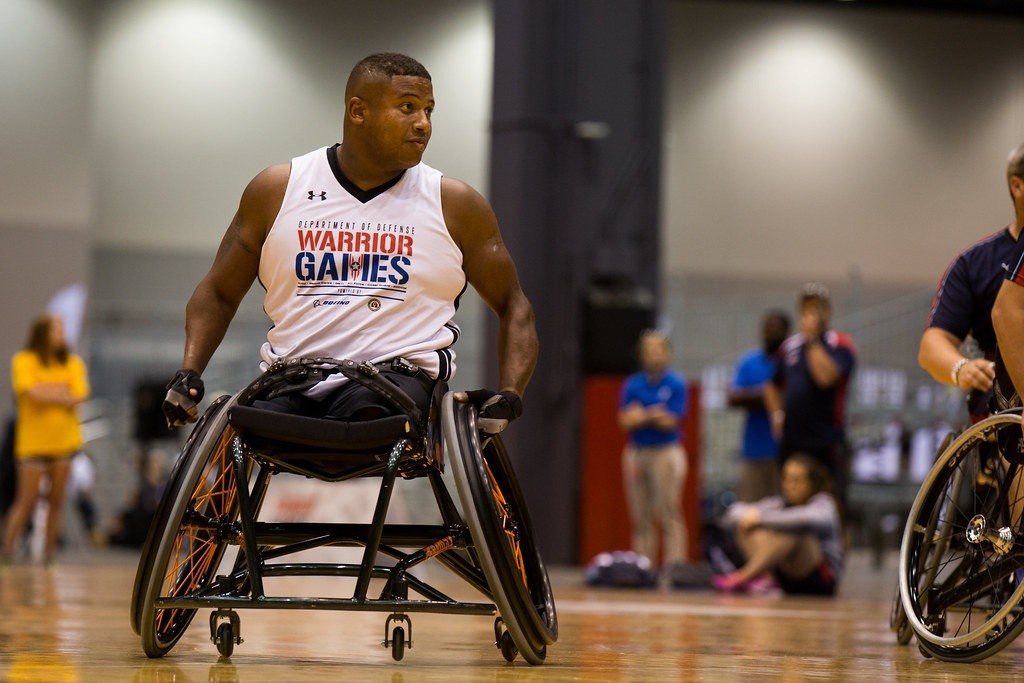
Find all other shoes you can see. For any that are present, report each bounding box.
[711,570,743,590]
[746,575,773,595]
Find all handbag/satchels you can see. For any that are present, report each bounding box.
[586,551,658,589]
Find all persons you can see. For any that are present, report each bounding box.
[726,311,794,502]
[712,455,842,598]
[0,315,93,567]
[617,331,687,566]
[762,288,855,479]
[163,54,537,435]
[916,145,1024,535]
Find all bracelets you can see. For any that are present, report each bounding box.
[951,359,969,385]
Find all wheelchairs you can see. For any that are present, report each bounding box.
[890,385,1024,664]
[130,353,558,666]
